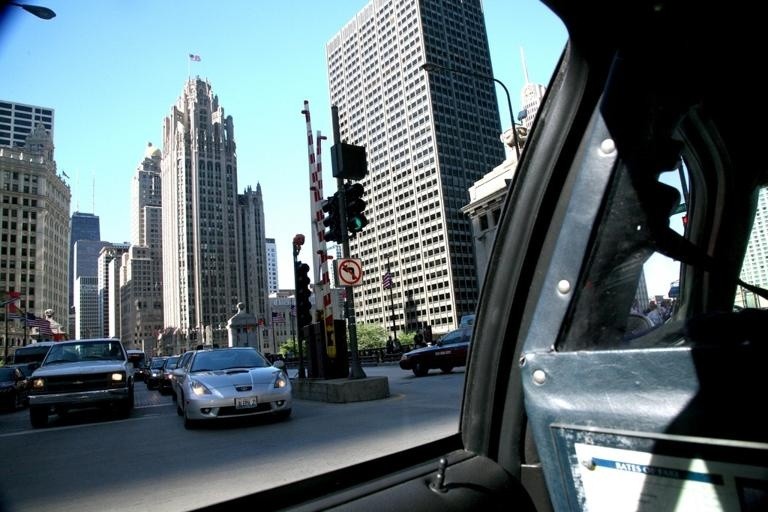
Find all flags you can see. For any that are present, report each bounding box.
[0,290,65,342]
[382,271,393,290]
[188,53,201,62]
[237,304,296,343]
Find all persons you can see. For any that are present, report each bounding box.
[386,329,442,362]
[631,297,680,328]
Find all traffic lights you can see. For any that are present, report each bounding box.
[322,183,368,242]
[299,263,312,326]
[331,143,367,180]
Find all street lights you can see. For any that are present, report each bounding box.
[0,2,56,19]
[421,61,520,159]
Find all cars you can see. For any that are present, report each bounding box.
[0,338,293,429]
[399,325,473,377]
